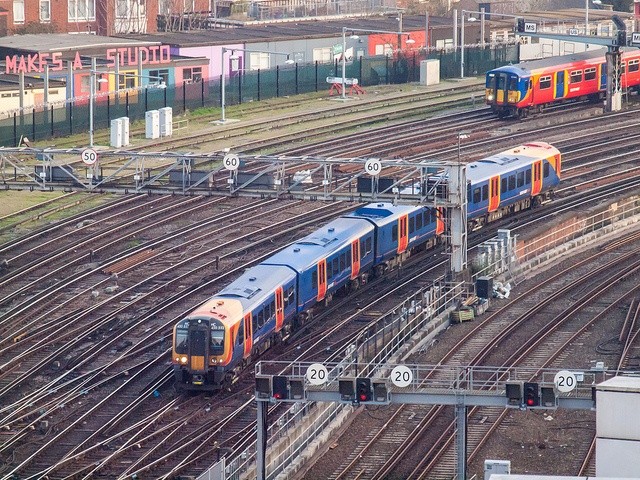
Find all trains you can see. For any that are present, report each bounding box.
[172,142,561,389]
[485,47,640,119]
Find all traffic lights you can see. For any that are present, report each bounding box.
[373,381,387,402]
[290,379,303,398]
[356,378,371,402]
[518,19,524,32]
[255,376,271,398]
[506,384,521,405]
[541,387,555,406]
[339,380,354,401]
[618,31,626,46]
[273,376,287,398]
[524,382,538,406]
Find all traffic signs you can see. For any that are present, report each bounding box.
[391,365,412,387]
[365,158,381,175]
[306,364,328,385]
[554,370,576,392]
[223,154,239,170]
[81,148,97,165]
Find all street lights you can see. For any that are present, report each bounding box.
[341,27,416,99]
[221,47,294,121]
[90,70,167,147]
